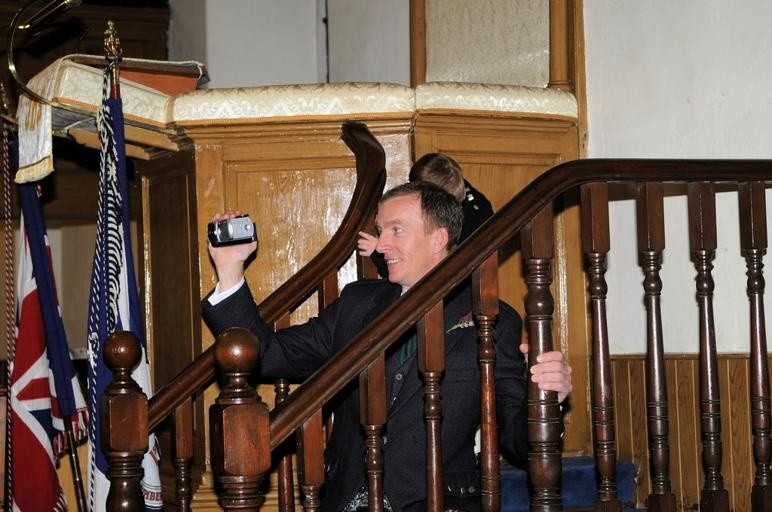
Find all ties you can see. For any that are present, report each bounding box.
[396,332,419,365]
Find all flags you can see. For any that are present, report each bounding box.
[84,58,168,511]
[6,176,93,511]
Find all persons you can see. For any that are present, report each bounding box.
[197,177,576,511]
[352,152,502,277]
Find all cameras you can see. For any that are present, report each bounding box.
[207,214,258,247]
